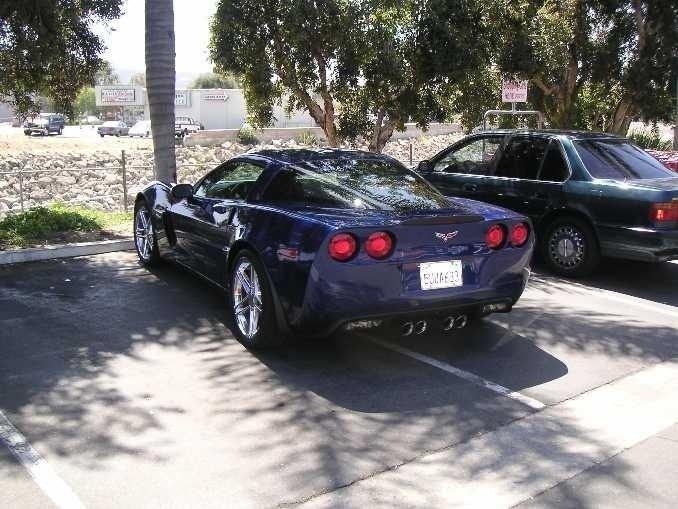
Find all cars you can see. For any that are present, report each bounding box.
[403,125,677,279]
[74,106,150,138]
[641,147,677,173]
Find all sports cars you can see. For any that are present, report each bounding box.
[125,146,541,352]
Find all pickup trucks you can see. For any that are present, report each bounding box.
[174,115,203,137]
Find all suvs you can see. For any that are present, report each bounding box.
[21,111,66,137]
